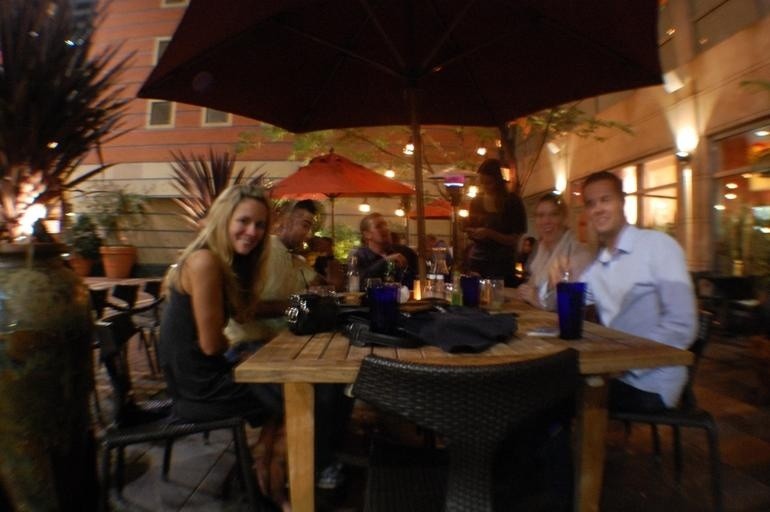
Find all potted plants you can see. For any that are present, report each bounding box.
[95,183,151,278]
[67,212,94,276]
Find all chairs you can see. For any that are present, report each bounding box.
[608,309,720,511]
[351,347,579,512]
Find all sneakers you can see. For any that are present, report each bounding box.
[245,464,287,512]
[315,460,350,491]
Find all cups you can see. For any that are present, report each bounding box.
[366,283,401,336]
[366,278,382,288]
[305,285,327,296]
[554,281,586,340]
[323,285,336,298]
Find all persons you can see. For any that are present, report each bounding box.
[513,192,585,313]
[303,227,536,294]
[221,194,348,494]
[342,213,419,292]
[498,168,702,449]
[457,157,530,288]
[154,181,293,511]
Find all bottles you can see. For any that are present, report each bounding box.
[424,274,509,315]
[385,259,399,286]
[425,247,450,291]
[346,255,361,293]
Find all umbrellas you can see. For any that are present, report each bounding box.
[403,199,469,226]
[260,151,413,261]
[132,1,672,308]
